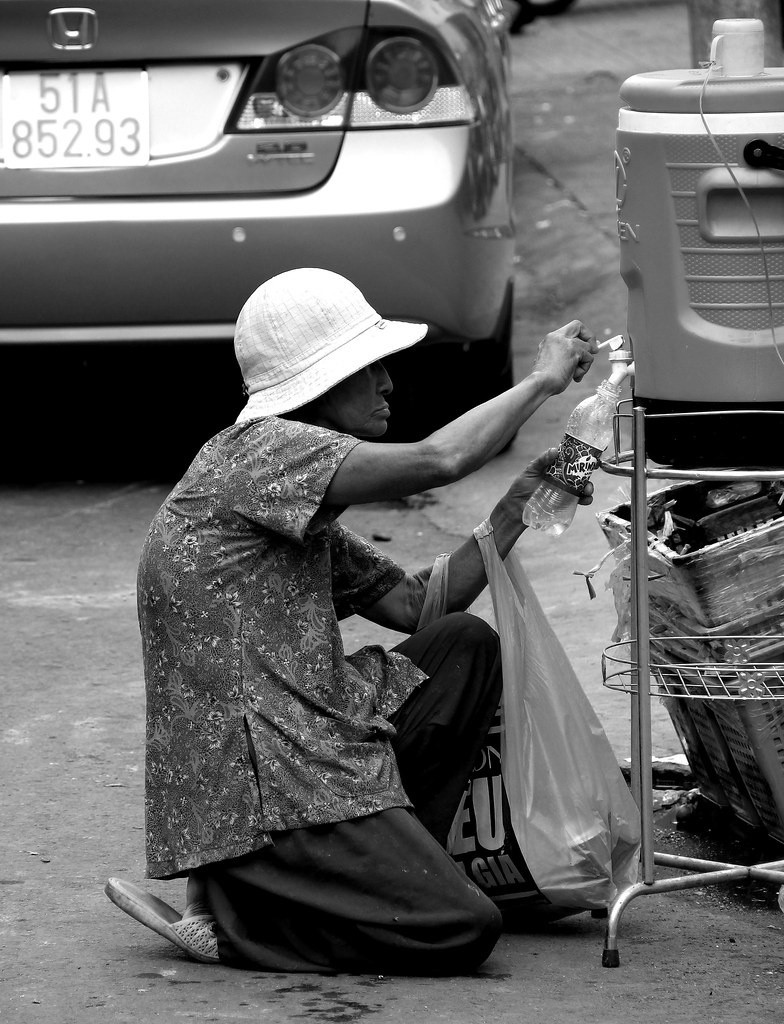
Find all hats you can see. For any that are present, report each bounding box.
[233,268,427,423]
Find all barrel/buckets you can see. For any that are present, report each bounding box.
[613,68,784,401]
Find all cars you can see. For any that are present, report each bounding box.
[0,0,516,462]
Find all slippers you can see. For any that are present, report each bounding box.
[103,878,219,961]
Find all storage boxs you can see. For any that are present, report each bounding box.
[596,475,784,846]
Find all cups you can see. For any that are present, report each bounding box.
[711,18,765,76]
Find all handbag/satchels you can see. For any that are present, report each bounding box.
[415,518,644,931]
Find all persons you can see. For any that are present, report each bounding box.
[103,267,598,980]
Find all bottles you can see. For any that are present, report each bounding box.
[521,378,621,537]
